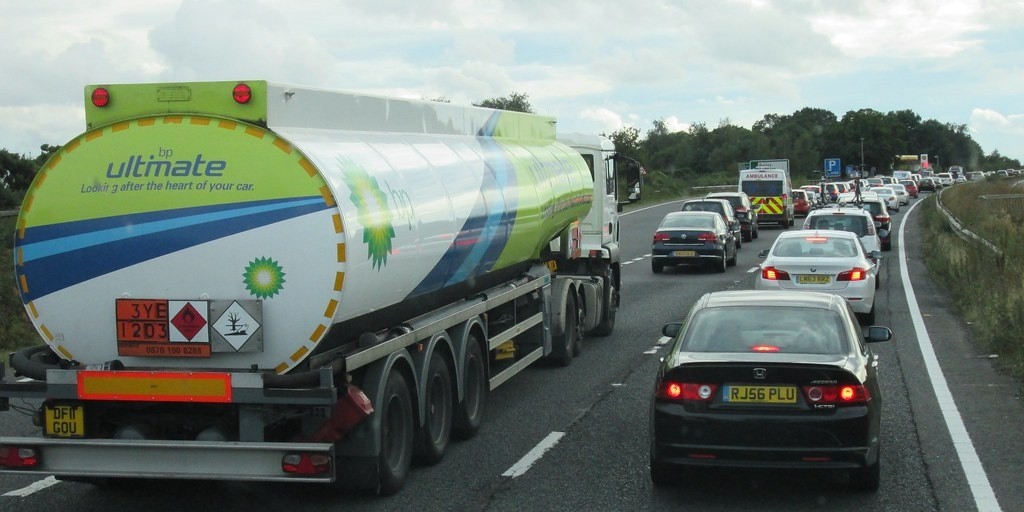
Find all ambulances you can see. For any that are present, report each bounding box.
[739,167,794,226]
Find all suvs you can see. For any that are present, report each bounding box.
[707,192,758,242]
[680,199,741,251]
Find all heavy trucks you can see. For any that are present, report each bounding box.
[0,80,646,495]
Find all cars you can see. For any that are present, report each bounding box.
[650,289,891,490]
[757,229,876,325]
[791,167,1024,216]
[803,208,882,286]
[653,211,738,272]
[839,199,893,253]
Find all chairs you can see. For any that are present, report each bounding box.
[784,241,804,256]
[831,240,851,257]
[819,220,830,229]
[704,321,746,350]
[835,223,843,230]
[847,220,860,233]
[793,327,829,353]
[870,204,880,213]
